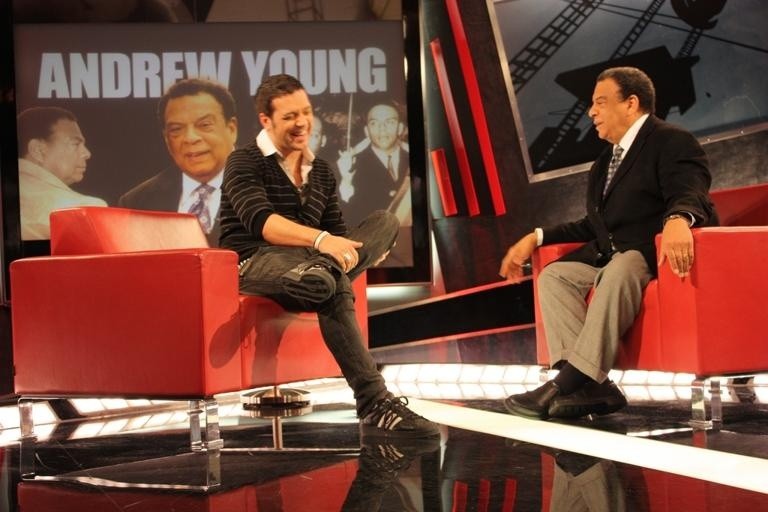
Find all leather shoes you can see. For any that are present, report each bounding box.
[503,381,563,421]
[548,380,627,419]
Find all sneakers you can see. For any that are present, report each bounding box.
[281,257,337,303]
[358,434,439,472]
[357,395,441,438]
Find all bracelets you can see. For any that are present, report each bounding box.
[664,213,691,224]
[314,229,330,250]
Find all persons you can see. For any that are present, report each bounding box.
[17,107,109,241]
[498,66,720,421]
[116,79,238,249]
[337,94,409,225]
[308,115,327,159]
[217,73,442,438]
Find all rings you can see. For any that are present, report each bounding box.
[682,251,690,259]
[343,252,351,263]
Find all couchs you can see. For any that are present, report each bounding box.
[538,448,768,511]
[15,455,365,512]
[530,183,768,430]
[9,205,371,455]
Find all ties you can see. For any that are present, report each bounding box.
[387,155,398,182]
[186,182,215,232]
[601,143,623,198]
